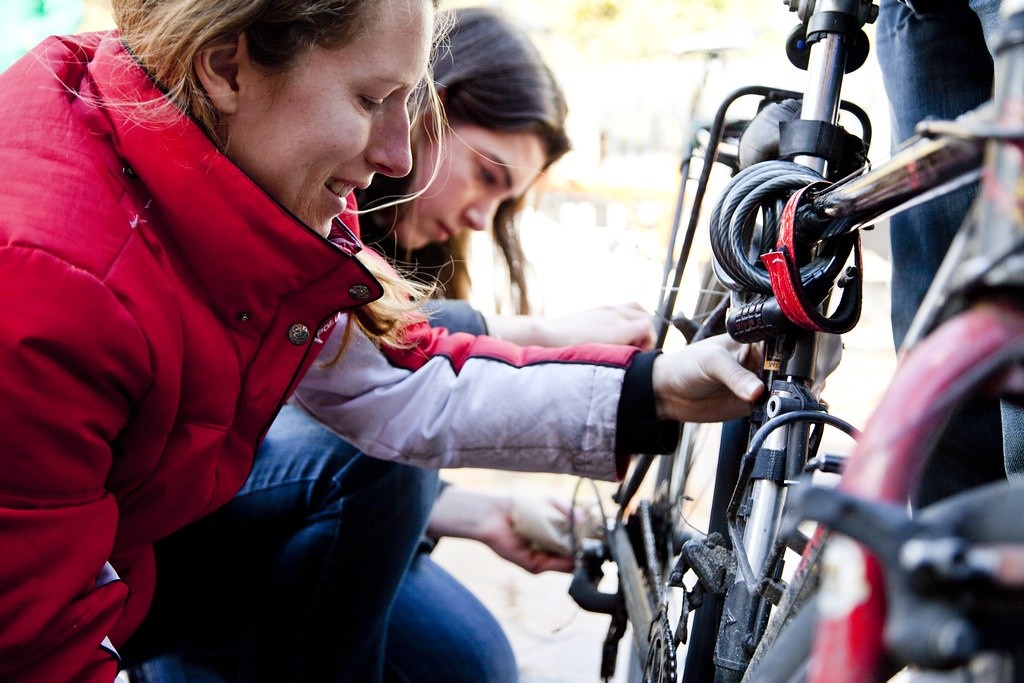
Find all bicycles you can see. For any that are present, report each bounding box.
[568,0,1024,683]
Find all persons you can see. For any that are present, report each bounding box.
[0,0,768,683]
[873,0,1024,514]
[380,6,657,683]
